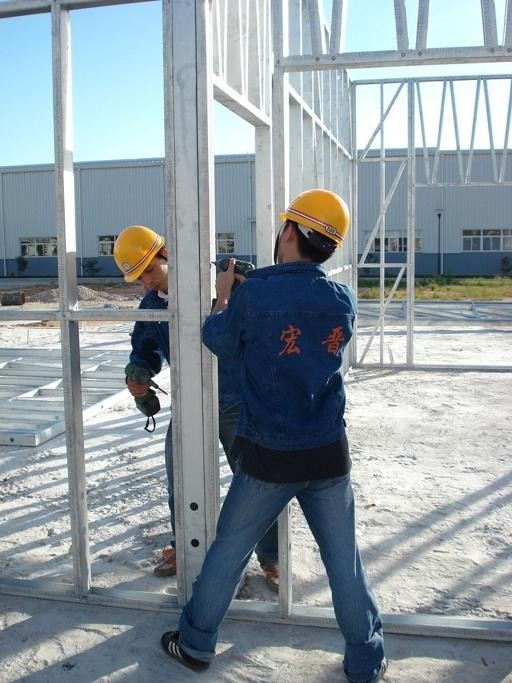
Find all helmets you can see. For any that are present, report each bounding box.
[278,188,351,248]
[112,225,164,284]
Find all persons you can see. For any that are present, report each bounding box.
[161,189,388,683]
[113,226,278,592]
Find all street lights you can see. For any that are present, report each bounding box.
[435,209,444,275]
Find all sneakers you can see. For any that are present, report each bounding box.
[154,546,176,577]
[160,630,211,674]
[346,655,388,683]
[265,570,278,591]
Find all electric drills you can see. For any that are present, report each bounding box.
[125,363,168,417]
[211,257,255,290]
